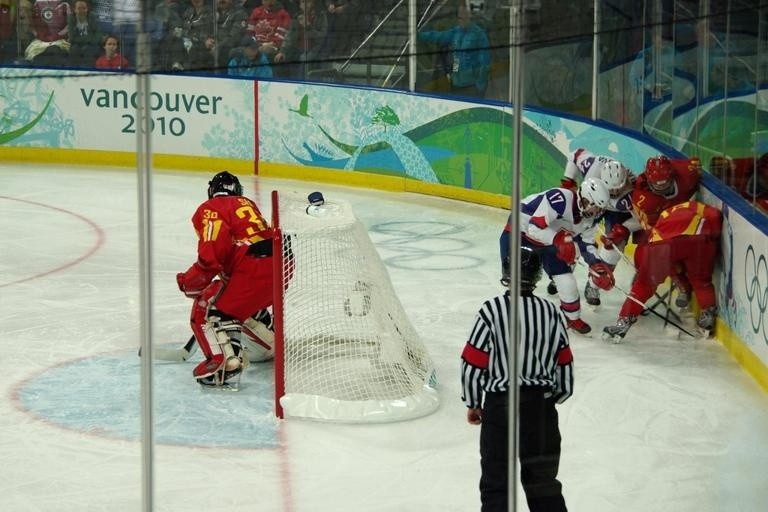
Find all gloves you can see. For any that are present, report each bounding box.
[589,262,615,291]
[600,224,630,249]
[553,230,576,265]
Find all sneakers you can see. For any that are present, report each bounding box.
[584,279,601,304]
[696,302,714,330]
[546,281,558,296]
[676,290,690,307]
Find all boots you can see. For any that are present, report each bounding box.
[560,300,591,334]
[603,317,637,338]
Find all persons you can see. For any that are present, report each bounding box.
[459,244,575,512]
[601,199,721,339]
[176,169,295,392]
[632,153,701,308]
[706,150,767,218]
[547,146,635,309]
[0,0,380,83]
[630,16,749,135]
[420,1,499,101]
[492,175,616,337]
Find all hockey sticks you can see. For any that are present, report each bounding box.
[138,335,199,361]
[575,224,699,339]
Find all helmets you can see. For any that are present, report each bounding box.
[208,173,242,197]
[503,247,540,286]
[578,156,672,219]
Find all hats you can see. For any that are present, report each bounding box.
[242,35,261,48]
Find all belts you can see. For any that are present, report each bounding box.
[485,391,552,406]
